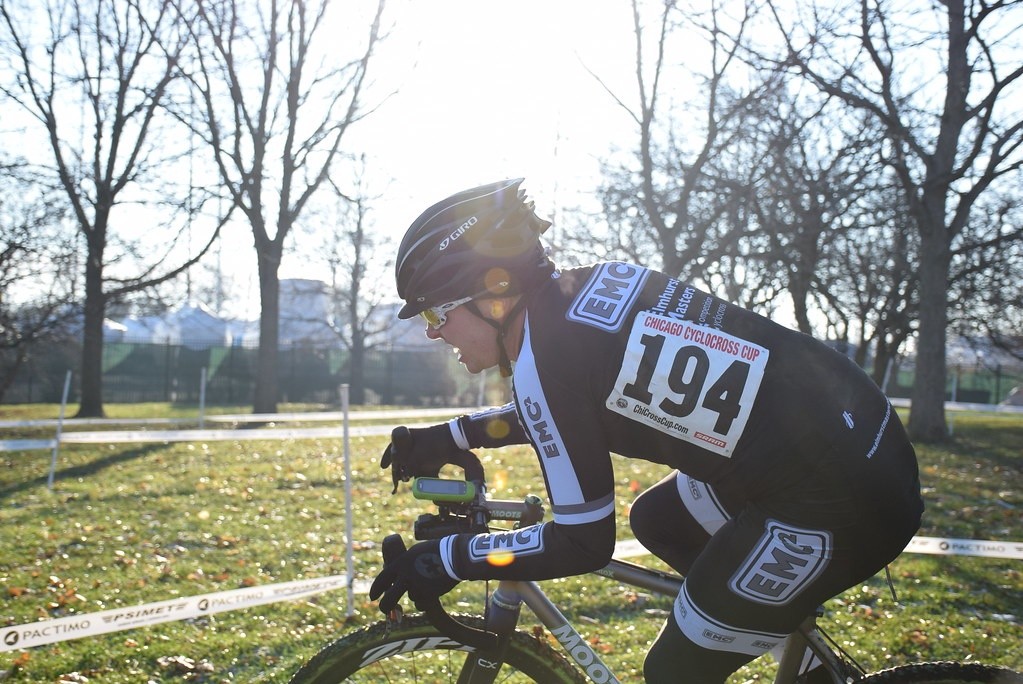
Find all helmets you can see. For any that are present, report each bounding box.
[395,178,555,319]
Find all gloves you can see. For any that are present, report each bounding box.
[381,414,477,482]
[369,533,476,614]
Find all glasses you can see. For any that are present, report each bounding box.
[419,282,509,329]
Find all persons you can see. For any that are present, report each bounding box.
[370,178,923,684]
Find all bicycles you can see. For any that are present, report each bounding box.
[289,450,1023,684]
